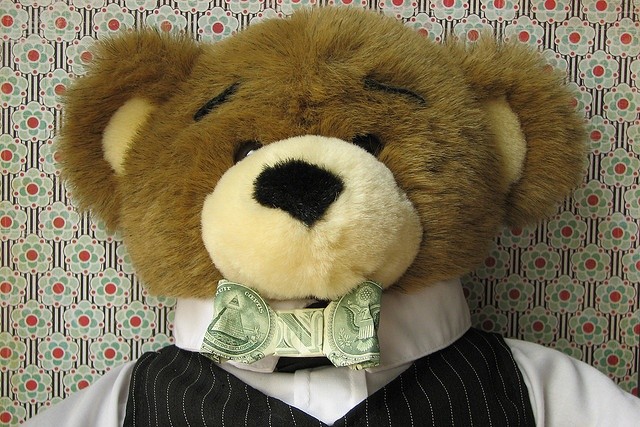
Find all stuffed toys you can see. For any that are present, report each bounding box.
[20,5,640,427]
